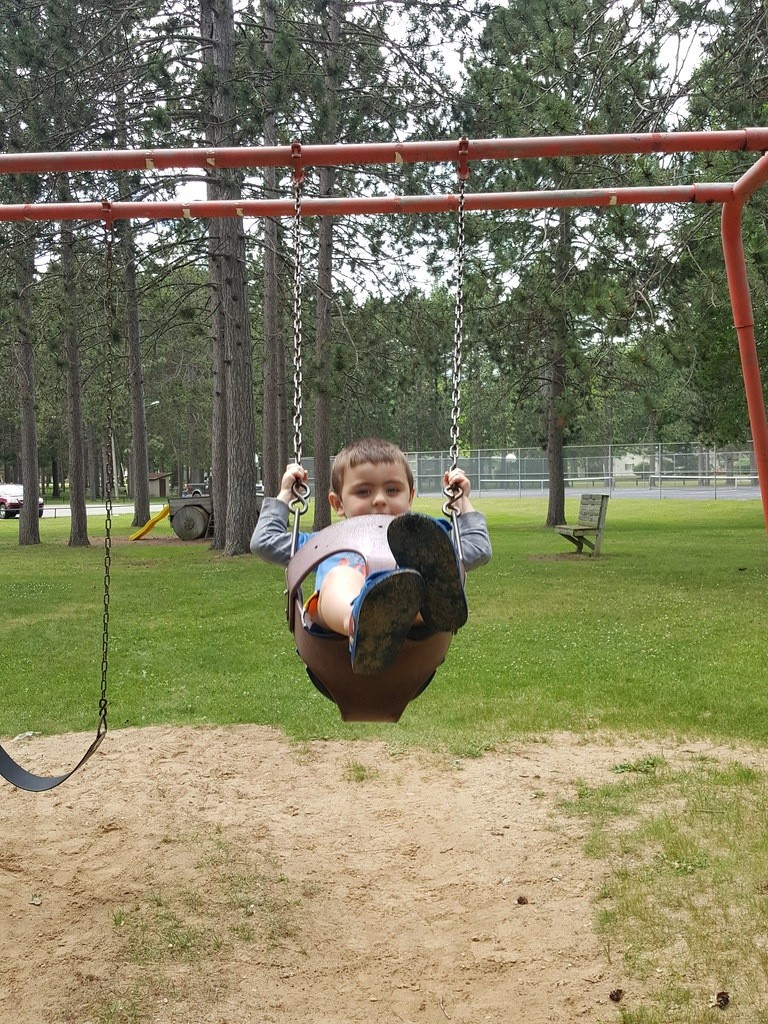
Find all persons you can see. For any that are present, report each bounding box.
[249,437,493,703]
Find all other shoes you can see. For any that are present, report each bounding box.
[349,568,424,678]
[387,513,468,633]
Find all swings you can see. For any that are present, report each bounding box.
[280,159,474,722]
[1,218,117,793]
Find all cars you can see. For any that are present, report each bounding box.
[0,478,45,520]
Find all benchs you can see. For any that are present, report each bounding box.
[555,494,610,557]
